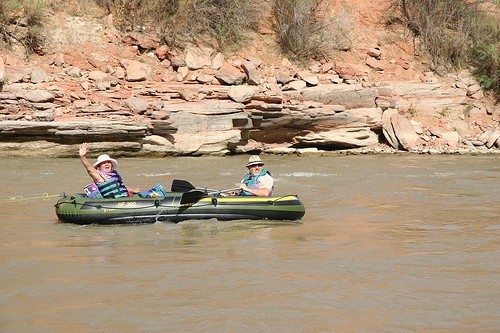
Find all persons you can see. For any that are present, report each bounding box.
[79,142,141,199]
[230,155,273,197]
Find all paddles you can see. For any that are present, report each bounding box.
[180,188,241,204]
[171,179,217,192]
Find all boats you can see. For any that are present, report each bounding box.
[54,192,306,224]
